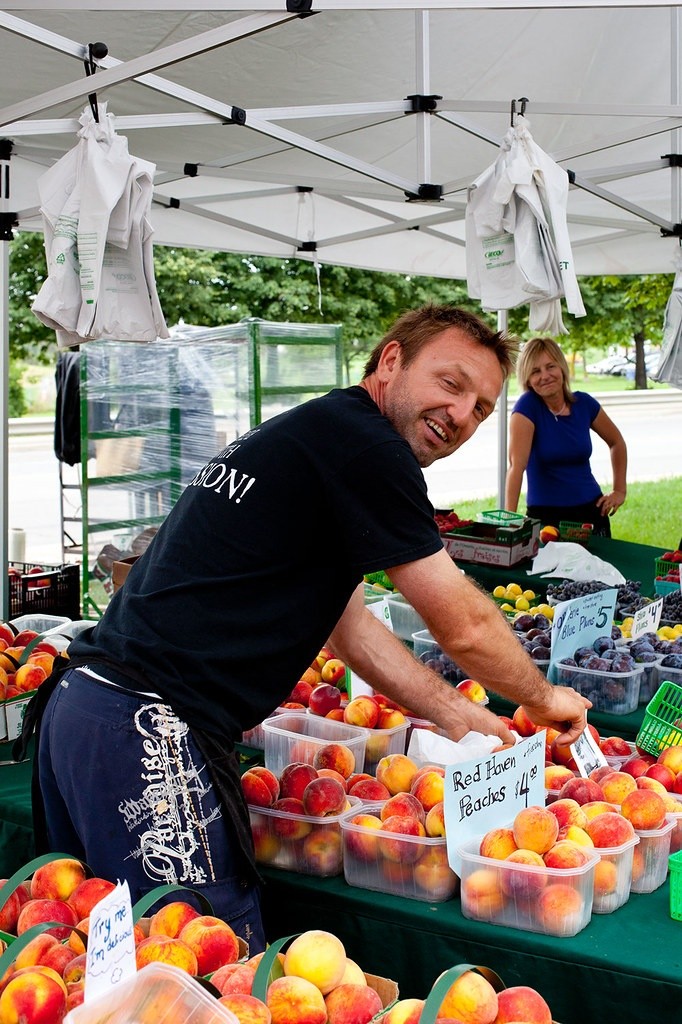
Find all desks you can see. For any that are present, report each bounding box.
[452,535,674,608]
[2,732,681,1024]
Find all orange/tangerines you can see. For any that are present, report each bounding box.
[493,582,554,619]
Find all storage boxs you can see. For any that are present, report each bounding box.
[598,736,638,765]
[203,950,401,1024]
[346,596,395,701]
[338,802,458,904]
[111,553,141,596]
[385,591,432,643]
[482,509,523,526]
[43,619,100,653]
[654,556,680,578]
[562,537,590,551]
[457,836,601,937]
[410,626,468,683]
[442,517,542,570]
[5,689,40,740]
[632,814,678,895]
[633,653,667,703]
[655,652,682,686]
[262,712,372,781]
[654,580,680,599]
[361,582,394,606]
[243,794,363,878]
[488,591,541,616]
[619,607,682,629]
[558,521,593,542]
[635,680,682,759]
[0,702,9,741]
[668,850,682,920]
[573,756,622,779]
[2,614,73,639]
[546,593,562,607]
[307,705,411,777]
[237,707,306,752]
[593,834,641,915]
[476,513,528,528]
[8,560,80,621]
[404,717,449,752]
[202,931,249,985]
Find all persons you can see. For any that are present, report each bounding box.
[504,338,629,537]
[15,301,592,957]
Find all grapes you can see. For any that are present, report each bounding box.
[546,579,681,623]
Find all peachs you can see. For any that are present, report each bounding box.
[540,526,560,545]
[0,624,682,1024]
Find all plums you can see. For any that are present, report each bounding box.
[418,614,682,711]
